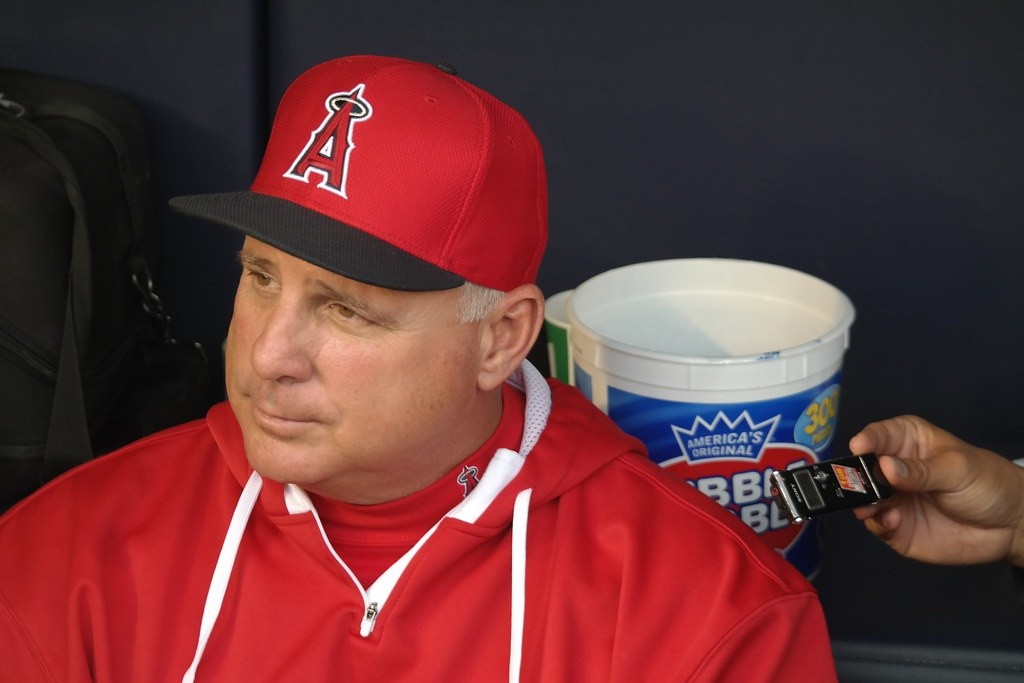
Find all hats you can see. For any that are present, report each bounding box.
[169,54,550,294]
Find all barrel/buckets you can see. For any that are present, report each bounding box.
[543,258,855,585]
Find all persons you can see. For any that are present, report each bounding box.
[0,53,835,681]
[848,414,1024,566]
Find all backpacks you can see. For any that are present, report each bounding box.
[0,69,205,512]
